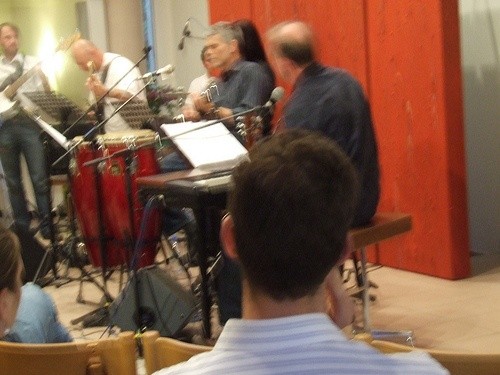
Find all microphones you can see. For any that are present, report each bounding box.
[139,64,175,79]
[256,86,284,122]
[178,21,191,49]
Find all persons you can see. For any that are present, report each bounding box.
[72,38,147,134]
[168,21,270,152]
[210,22,382,339]
[0,21,56,240]
[0,226,74,343]
[149,127,450,375]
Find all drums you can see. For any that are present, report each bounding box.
[68,135,125,270]
[97,129,161,271]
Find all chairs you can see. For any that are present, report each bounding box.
[0,330,139,375]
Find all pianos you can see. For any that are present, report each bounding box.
[135,154,247,346]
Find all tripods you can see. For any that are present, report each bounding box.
[33,54,157,305]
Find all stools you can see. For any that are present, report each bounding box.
[347,216,413,339]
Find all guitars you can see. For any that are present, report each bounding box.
[236,110,263,150]
[0,27,82,123]
[179,78,219,122]
